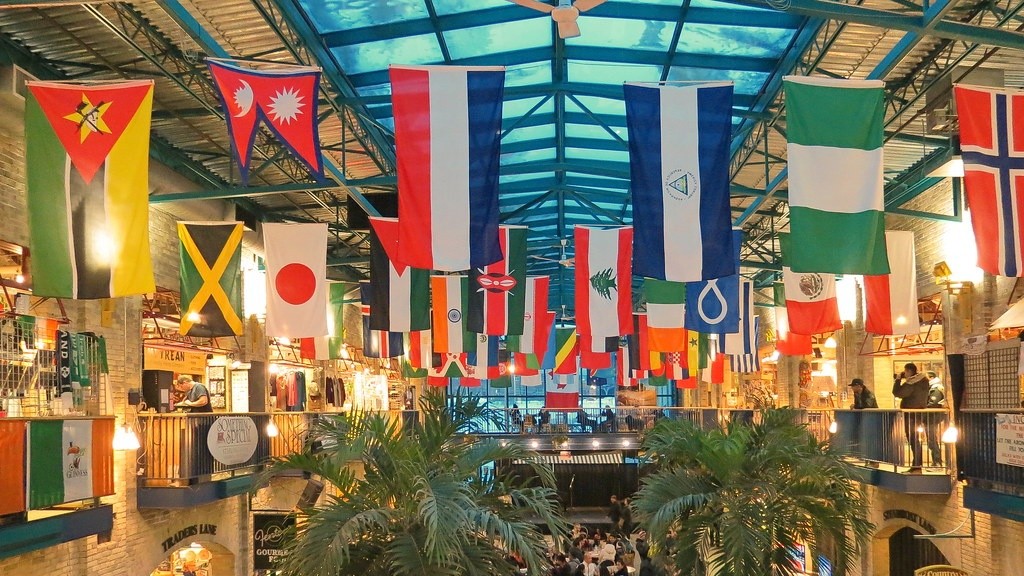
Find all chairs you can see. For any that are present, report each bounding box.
[511,414,522,434]
[542,414,552,433]
[607,413,618,432]
[523,414,535,433]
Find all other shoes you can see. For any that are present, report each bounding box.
[931,459,942,466]
[865,462,879,468]
[901,468,922,474]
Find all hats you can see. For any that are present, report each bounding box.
[639,530,646,538]
[848,378,863,386]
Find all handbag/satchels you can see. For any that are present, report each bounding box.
[936,398,947,424]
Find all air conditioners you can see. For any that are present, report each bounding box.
[109,294,142,451]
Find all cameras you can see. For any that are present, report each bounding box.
[893,372,906,379]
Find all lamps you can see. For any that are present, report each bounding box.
[933,261,970,295]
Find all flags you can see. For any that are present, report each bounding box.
[25,81,156,298]
[782,76,890,276]
[771,231,843,355]
[620,84,736,281]
[545,369,579,412]
[261,221,329,339]
[390,65,505,269]
[952,85,1024,278]
[863,230,919,336]
[300,216,761,389]
[178,222,243,336]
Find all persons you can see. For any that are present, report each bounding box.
[893,363,930,472]
[511,404,524,432]
[178,375,213,485]
[607,495,631,537]
[537,406,550,433]
[709,506,724,547]
[577,406,596,432]
[601,405,613,430]
[921,371,946,466]
[848,378,880,468]
[500,522,675,576]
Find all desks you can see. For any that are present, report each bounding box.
[584,550,637,576]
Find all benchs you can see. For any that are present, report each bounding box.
[567,424,599,432]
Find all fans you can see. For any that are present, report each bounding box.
[531,240,575,268]
[555,305,575,320]
[506,0,608,38]
